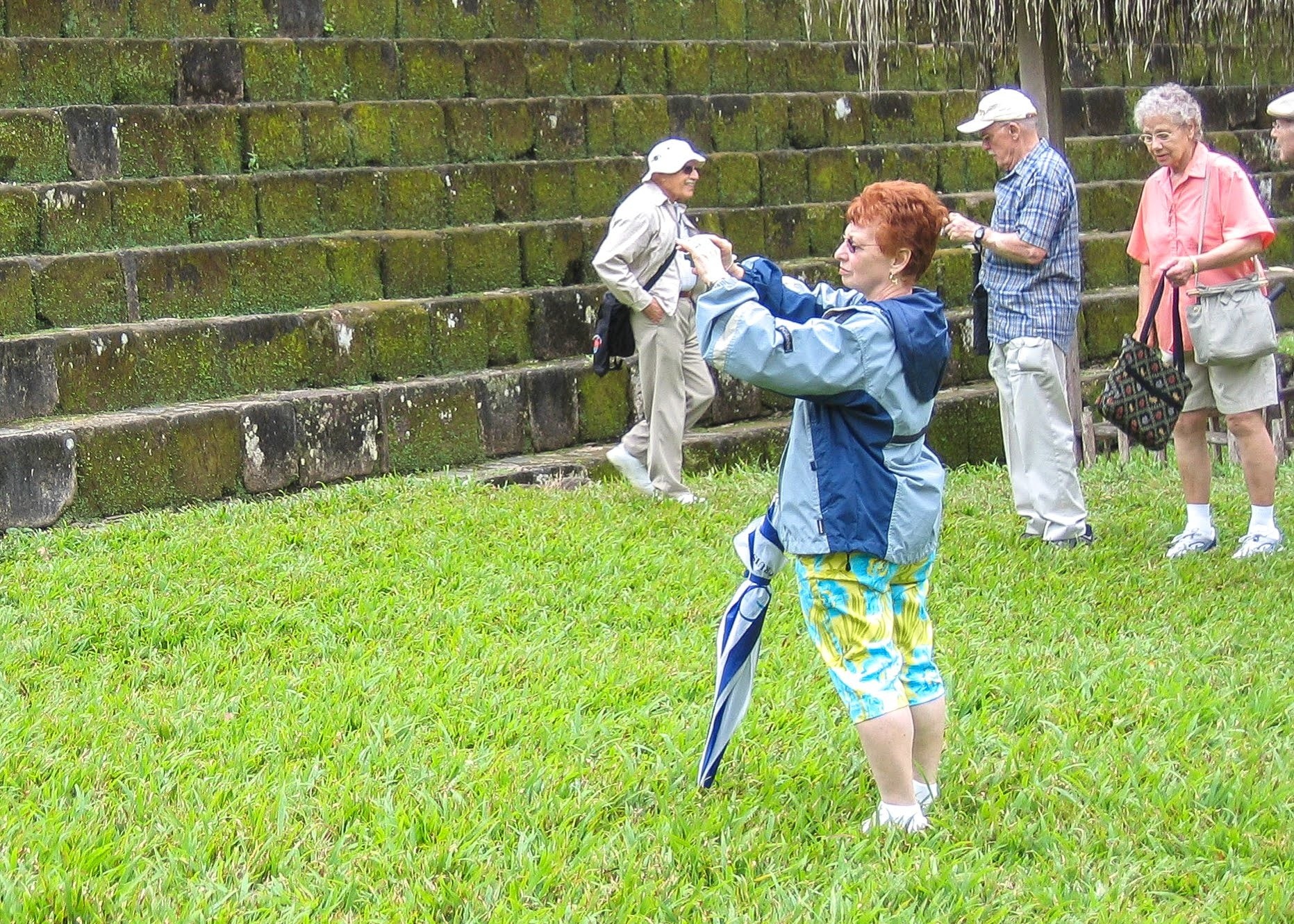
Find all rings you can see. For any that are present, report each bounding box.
[695,244,701,248]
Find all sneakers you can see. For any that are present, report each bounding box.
[655,489,705,504]
[605,443,655,493]
[1043,525,1093,549]
[1231,529,1282,558]
[1165,526,1217,557]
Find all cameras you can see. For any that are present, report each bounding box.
[684,252,695,268]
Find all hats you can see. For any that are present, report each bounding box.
[956,87,1037,134]
[1266,91,1294,119]
[641,138,705,182]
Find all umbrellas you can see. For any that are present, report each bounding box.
[696,491,784,794]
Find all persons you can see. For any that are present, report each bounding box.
[1267,91,1294,162]
[592,138,715,507]
[1127,83,1283,558]
[675,180,948,834]
[940,87,1093,549]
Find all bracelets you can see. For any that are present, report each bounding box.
[1190,255,1198,274]
[725,255,734,272]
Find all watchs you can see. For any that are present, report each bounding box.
[974,226,985,243]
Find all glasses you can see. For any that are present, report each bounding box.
[982,121,1020,143]
[1139,124,1181,144]
[682,166,701,175]
[839,234,905,253]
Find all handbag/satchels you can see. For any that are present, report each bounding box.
[1098,335,1193,451]
[593,294,635,377]
[1186,278,1278,365]
